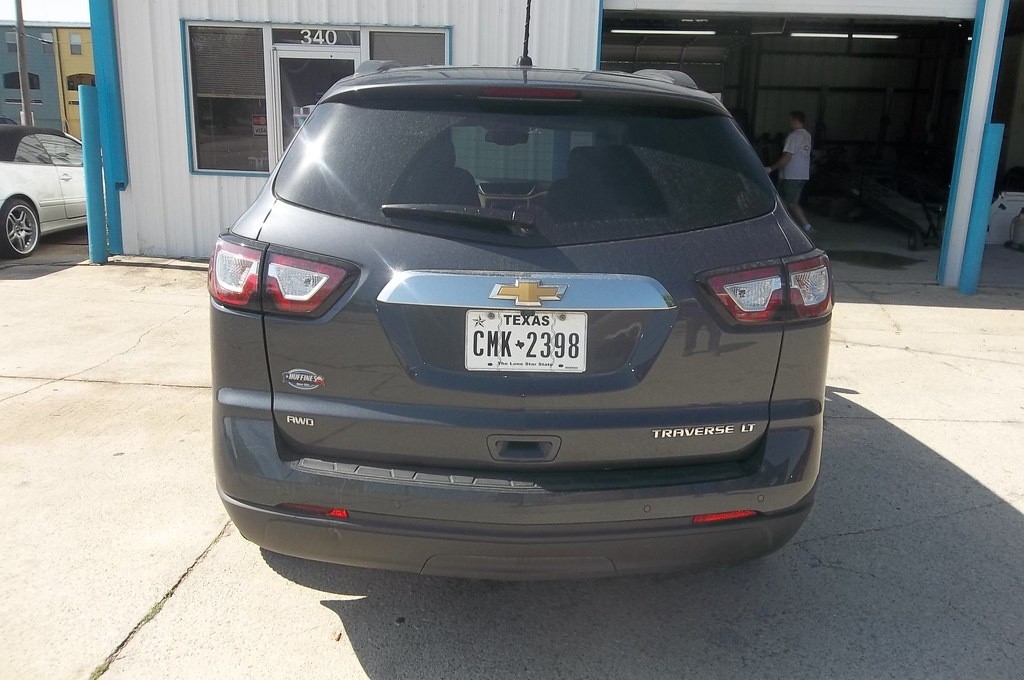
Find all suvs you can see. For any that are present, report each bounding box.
[204,58,835,572]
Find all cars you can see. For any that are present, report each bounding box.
[0,123,110,257]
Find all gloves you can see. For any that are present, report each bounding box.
[765,167,772,175]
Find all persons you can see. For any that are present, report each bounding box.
[681,304,722,357]
[765,111,814,233]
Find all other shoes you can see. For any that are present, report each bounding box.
[803,228,816,241]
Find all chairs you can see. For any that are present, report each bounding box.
[368,131,482,208]
[542,143,671,223]
